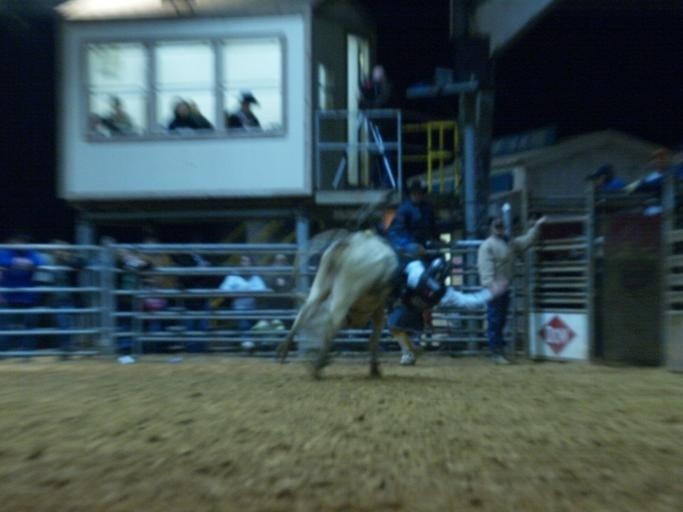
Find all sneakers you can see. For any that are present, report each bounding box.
[492,353,512,365]
[400,349,415,365]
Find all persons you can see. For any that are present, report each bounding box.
[0,147,682,365]
[92,90,262,129]
[361,66,396,188]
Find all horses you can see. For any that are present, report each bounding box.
[273,185,400,382]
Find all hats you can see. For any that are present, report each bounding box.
[405,181,427,196]
